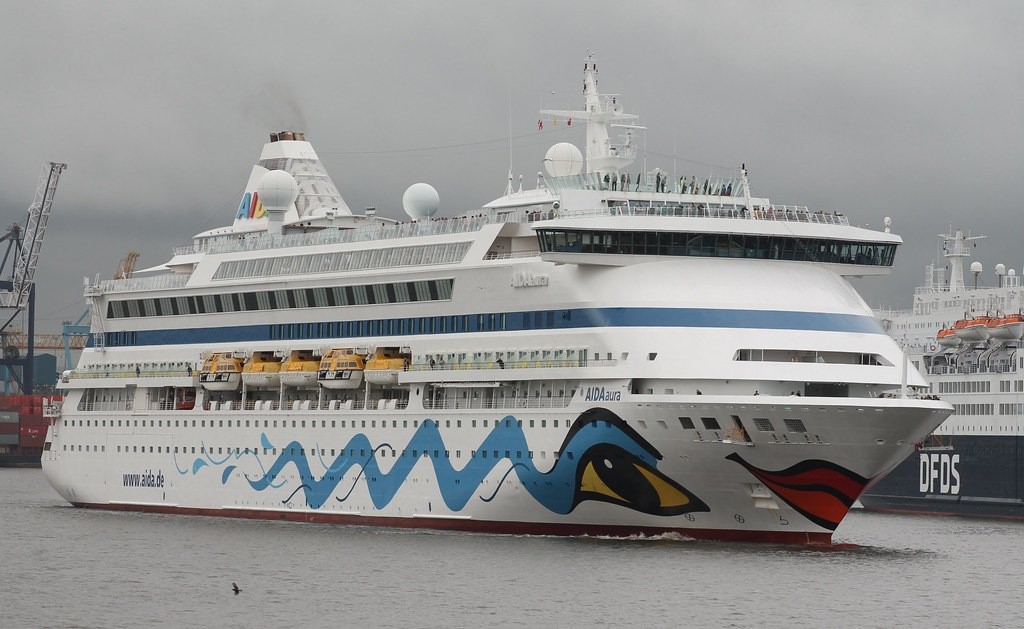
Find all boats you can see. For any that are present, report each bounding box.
[199,372,242,391]
[936,328,961,347]
[278,371,316,388]
[363,369,401,384]
[241,373,280,387]
[317,369,363,390]
[953,324,990,343]
[991,322,1024,342]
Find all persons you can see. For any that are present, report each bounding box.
[791,392,794,395]
[603,172,731,197]
[867,247,884,265]
[439,357,445,370]
[497,358,504,369]
[376,202,846,238]
[403,358,409,372]
[697,390,702,395]
[186,366,193,377]
[754,391,759,396]
[136,366,140,378]
[429,358,435,371]
[797,391,801,397]
[880,393,941,402]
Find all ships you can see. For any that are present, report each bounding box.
[857,223,1024,524]
[40,55,954,554]
